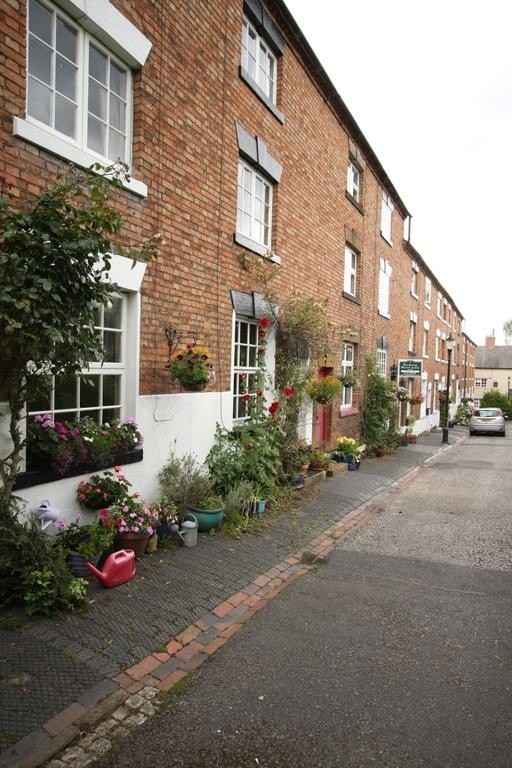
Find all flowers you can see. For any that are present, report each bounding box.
[397,386,425,405]
[307,371,343,405]
[30,412,141,479]
[52,466,177,556]
[171,344,217,388]
[335,435,361,460]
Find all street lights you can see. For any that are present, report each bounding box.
[443,332,455,444]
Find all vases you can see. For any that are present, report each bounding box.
[66,530,152,577]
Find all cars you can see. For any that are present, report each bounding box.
[469,407,509,436]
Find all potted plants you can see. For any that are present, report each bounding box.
[297,453,331,478]
[184,470,274,532]
